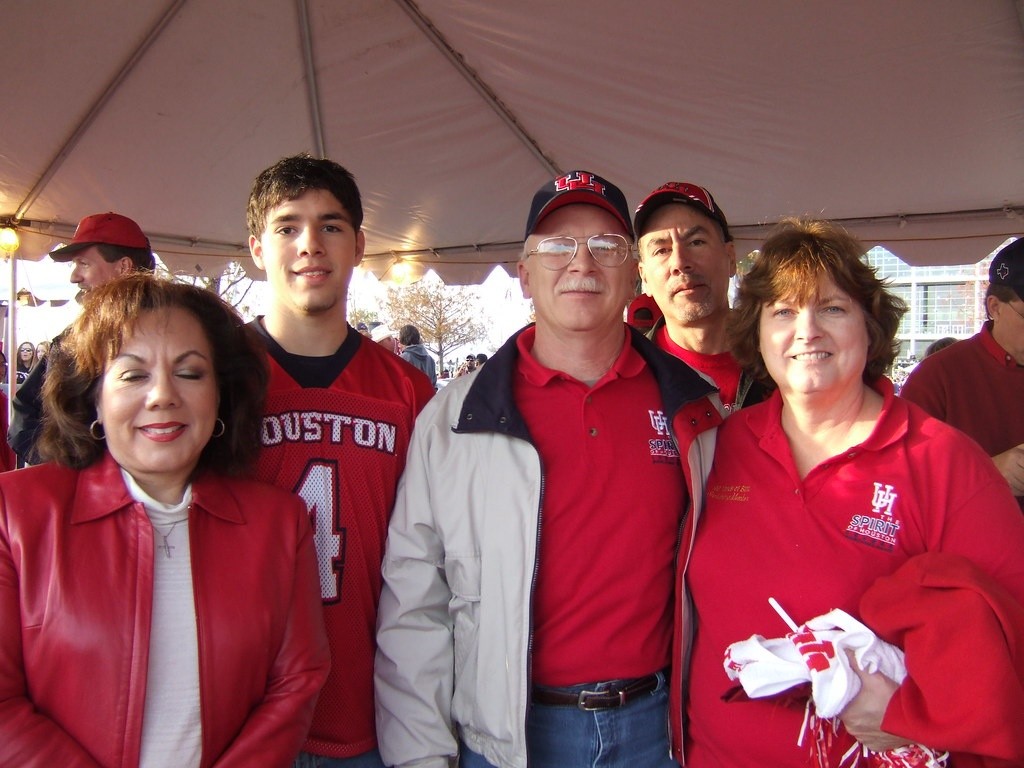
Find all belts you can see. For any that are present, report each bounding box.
[533,670,671,713]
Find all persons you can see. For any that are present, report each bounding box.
[457,355,476,378]
[681,216,1023,768]
[896,236,1023,509]
[16,342,36,384]
[29,342,51,373]
[634,181,740,411]
[8,212,155,467]
[398,325,439,393]
[0,270,330,768]
[0,352,17,474]
[231,152,437,768]
[374,169,728,768]
[474,354,487,370]
[371,325,396,353]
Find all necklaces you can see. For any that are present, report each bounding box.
[149,516,179,558]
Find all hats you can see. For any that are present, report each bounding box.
[524,169,635,244]
[467,354,476,360]
[49,212,151,262]
[371,324,396,343]
[633,182,730,241]
[357,323,368,330]
[989,237,1024,303]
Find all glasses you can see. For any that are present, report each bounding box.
[0,362,8,369]
[20,348,33,352]
[527,234,634,271]
[466,358,474,362]
